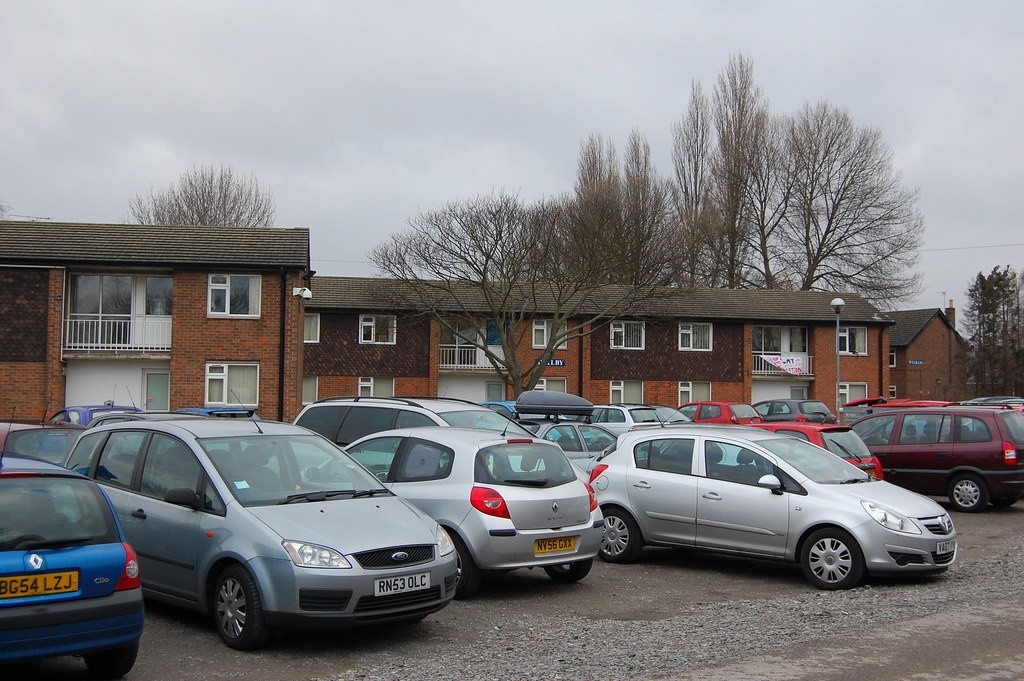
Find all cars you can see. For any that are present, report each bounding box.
[509,390,620,477]
[751,397,838,425]
[591,422,958,589]
[480,400,569,423]
[673,400,763,428]
[578,403,664,439]
[847,406,1024,512]
[0,457,147,679]
[80,412,204,431]
[48,402,142,431]
[0,420,91,469]
[840,394,1024,441]
[173,405,263,423]
[647,405,693,427]
[744,421,886,482]
[299,426,605,602]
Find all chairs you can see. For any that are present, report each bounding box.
[407,448,442,477]
[901,425,917,443]
[708,446,730,479]
[239,447,277,492]
[733,449,761,484]
[918,424,932,443]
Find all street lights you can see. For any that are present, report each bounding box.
[830,298,846,422]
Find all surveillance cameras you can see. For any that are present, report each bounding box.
[303,290,312,300]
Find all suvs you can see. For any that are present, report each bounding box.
[62,416,461,652]
[264,394,591,488]
